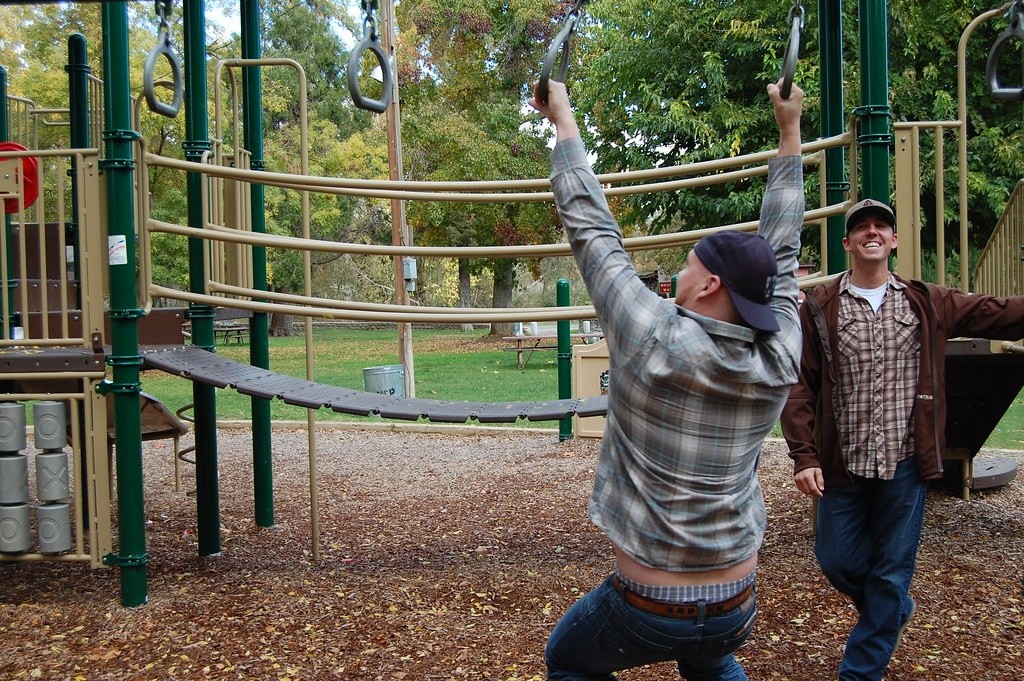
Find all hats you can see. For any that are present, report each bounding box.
[694,230,780,332]
[845,199,895,237]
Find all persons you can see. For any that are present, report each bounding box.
[525,78,804,681]
[781,198,1024,681]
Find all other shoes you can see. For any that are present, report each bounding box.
[890,597,916,654]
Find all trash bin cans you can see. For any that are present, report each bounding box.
[362,365,405,398]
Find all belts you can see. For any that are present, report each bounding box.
[611,574,754,619]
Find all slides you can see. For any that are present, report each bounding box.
[27,378,188,447]
